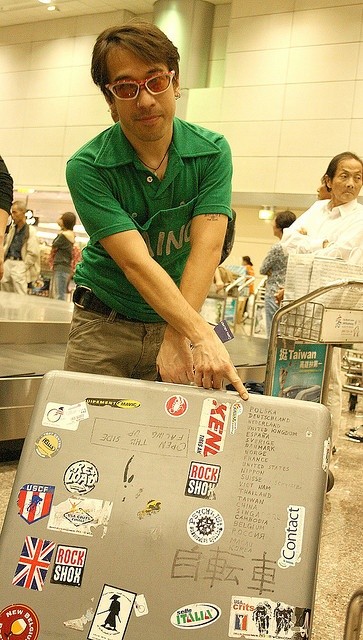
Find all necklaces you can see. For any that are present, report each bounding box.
[134,147,169,178]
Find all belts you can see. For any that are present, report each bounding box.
[70,285,146,324]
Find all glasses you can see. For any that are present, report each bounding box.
[104,70,176,103]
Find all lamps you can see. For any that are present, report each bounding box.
[258,206,274,219]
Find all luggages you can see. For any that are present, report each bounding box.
[1,369,331,640]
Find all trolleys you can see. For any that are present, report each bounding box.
[340,344,362,413]
[261,278,362,491]
[27,252,52,297]
[226,276,265,334]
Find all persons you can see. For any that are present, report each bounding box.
[62,15,252,402]
[259,210,297,339]
[238,255,256,319]
[280,151,362,456]
[1,154,14,280]
[1,200,41,296]
[51,211,77,302]
[273,172,333,306]
